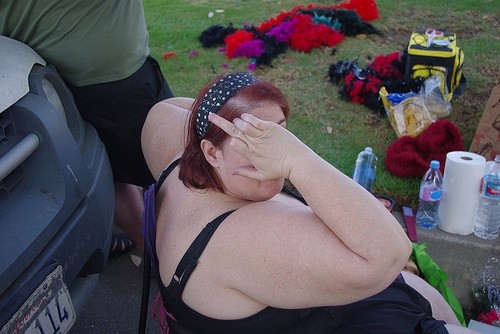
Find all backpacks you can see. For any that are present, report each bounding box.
[405,31,467,103]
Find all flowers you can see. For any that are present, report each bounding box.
[470,285,500,328]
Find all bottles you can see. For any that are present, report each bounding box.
[474,154,500,239]
[416,161,443,229]
[353,147,378,192]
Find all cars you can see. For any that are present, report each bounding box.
[0,35,115,334]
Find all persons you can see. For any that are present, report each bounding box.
[141,71,481,334]
[0,0,176,277]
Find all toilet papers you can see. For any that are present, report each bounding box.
[436,151,487,236]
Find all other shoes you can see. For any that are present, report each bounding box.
[109,233,136,259]
[129,254,156,277]
